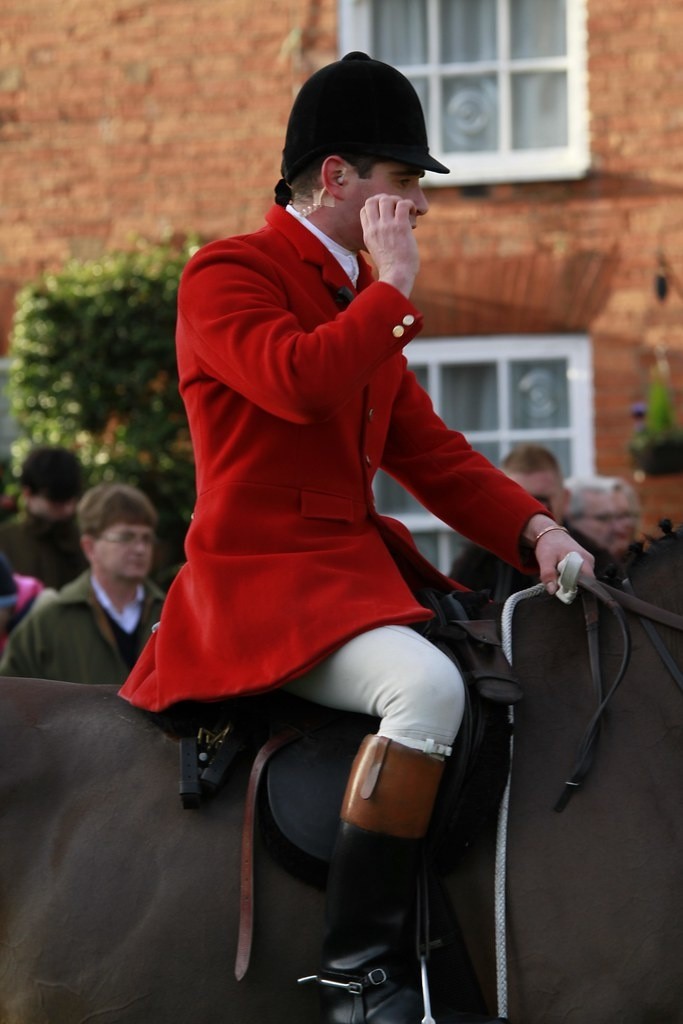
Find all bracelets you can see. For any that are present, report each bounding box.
[533,526,570,551]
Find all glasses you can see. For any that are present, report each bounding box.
[92,530,155,545]
[530,493,562,512]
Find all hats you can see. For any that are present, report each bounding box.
[281,51,450,185]
[21,446,86,502]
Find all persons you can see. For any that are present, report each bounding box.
[115,52,595,1024]
[0,488,166,686]
[0,553,59,661]
[0,449,93,593]
[447,445,622,618]
[562,476,640,552]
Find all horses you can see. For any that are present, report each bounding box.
[0,516,683,1024]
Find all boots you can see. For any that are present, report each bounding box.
[303,733,509,1024]
